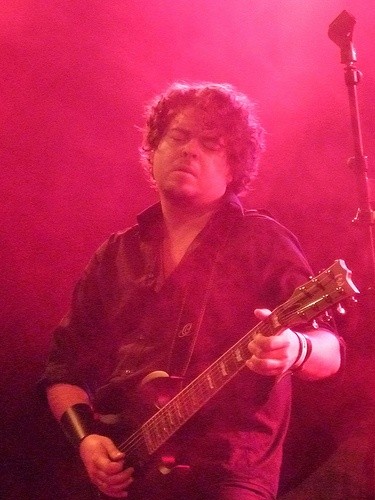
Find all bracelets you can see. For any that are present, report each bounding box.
[276,331,313,385]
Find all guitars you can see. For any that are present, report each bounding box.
[50,257,360,500]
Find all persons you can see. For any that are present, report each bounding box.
[34,83,347,500]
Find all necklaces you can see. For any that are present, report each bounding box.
[161,220,167,286]
